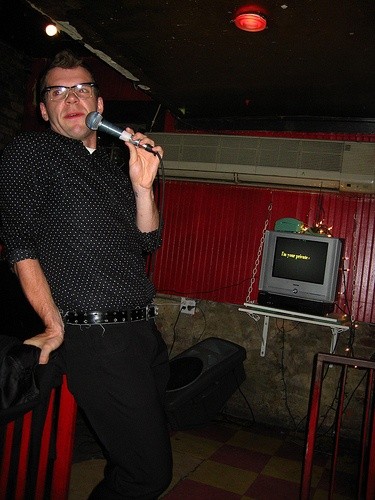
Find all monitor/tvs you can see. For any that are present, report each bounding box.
[258,230,341,303]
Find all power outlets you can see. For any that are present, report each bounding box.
[180,297,197,315]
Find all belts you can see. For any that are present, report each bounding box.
[61,304,158,325]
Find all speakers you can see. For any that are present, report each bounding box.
[168,337,247,428]
[97,100,164,175]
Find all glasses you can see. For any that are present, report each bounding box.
[40,82,94,101]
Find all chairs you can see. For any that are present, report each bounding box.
[0,342,76,500]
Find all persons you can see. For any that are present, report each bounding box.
[0,48,175,500]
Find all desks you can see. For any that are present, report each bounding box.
[304,344,375,500]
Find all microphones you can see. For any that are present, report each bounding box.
[85,111,158,155]
